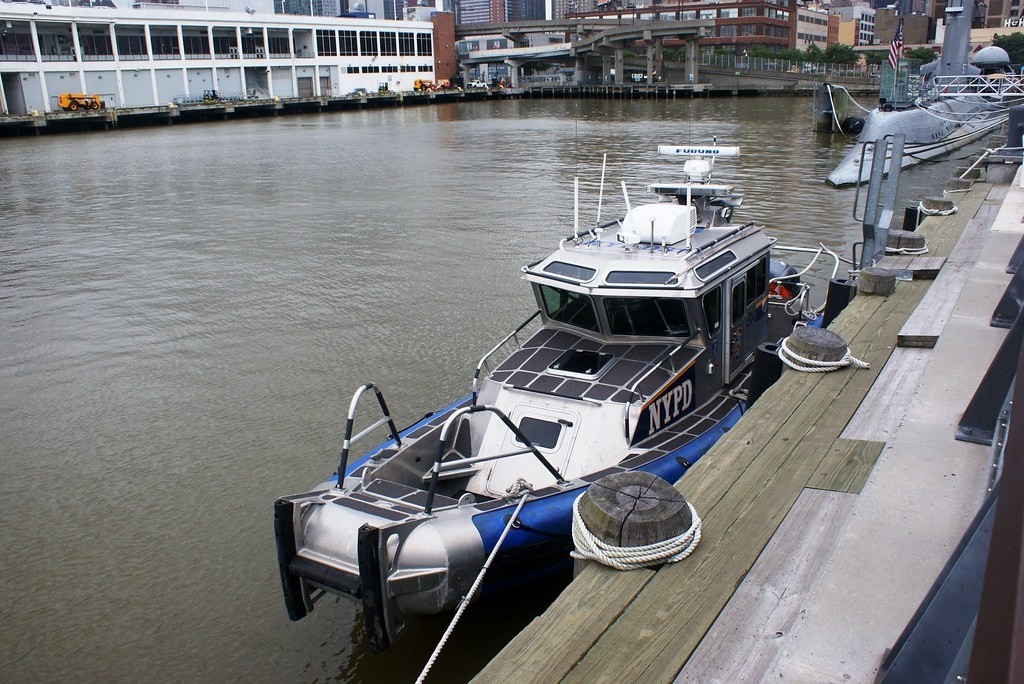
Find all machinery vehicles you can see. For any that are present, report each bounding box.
[57,92,101,112]
[413,78,436,92]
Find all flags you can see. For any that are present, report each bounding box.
[888,15,905,70]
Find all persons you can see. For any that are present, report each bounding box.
[248,89,258,100]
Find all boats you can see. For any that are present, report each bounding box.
[824,13,1011,191]
[272,134,845,655]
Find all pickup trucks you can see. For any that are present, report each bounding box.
[466,80,486,90]
